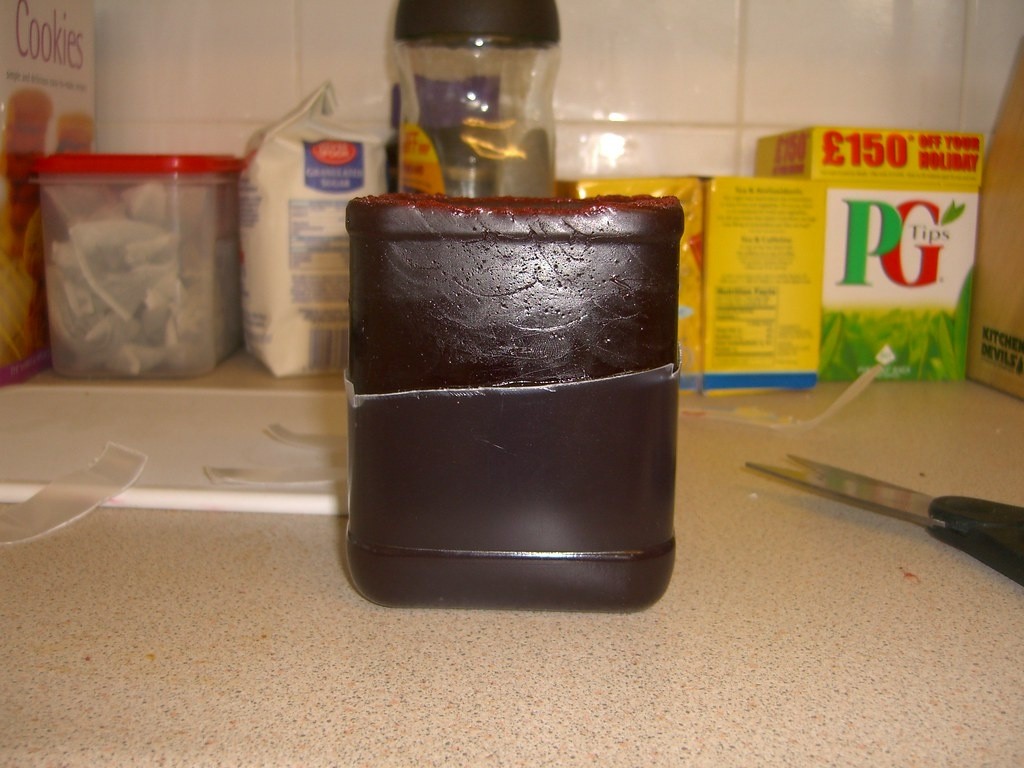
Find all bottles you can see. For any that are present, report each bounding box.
[392,0,562,203]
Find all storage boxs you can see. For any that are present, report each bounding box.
[0,0,96,388]
[552,35,1024,398]
[33,153,243,379]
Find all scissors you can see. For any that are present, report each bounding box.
[744,451,1024,589]
